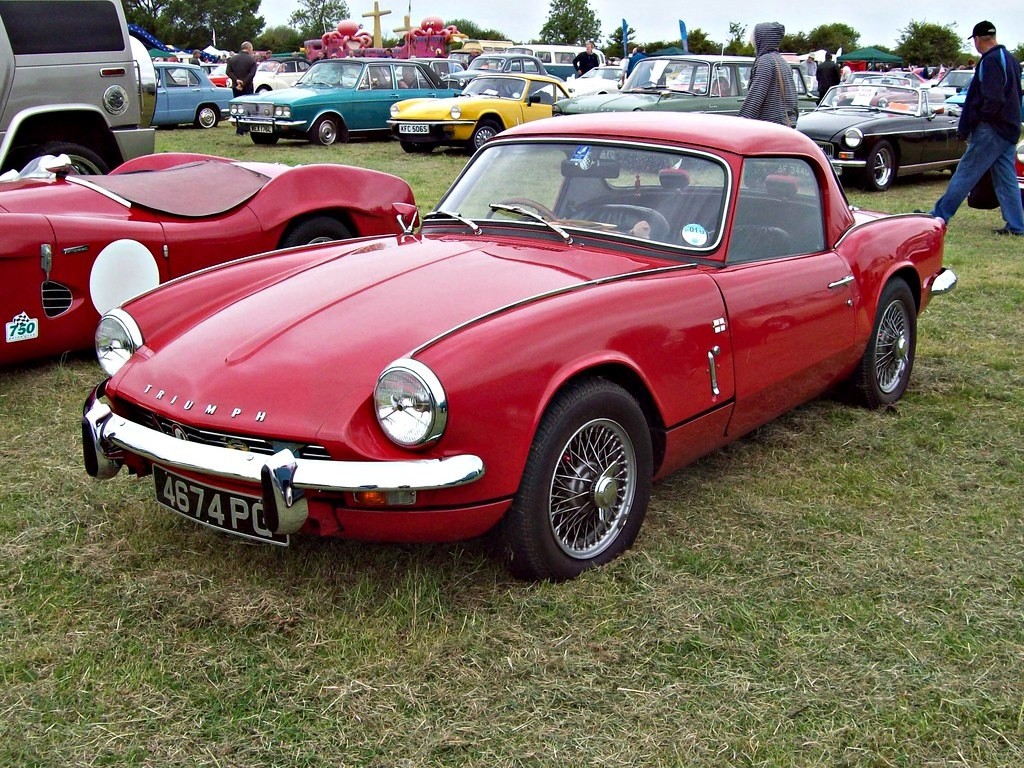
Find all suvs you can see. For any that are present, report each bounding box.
[0,0,156,180]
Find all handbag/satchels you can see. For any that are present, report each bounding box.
[967,164,1001,210]
[743,161,790,190]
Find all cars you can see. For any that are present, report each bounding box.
[167,54,314,95]
[150,62,234,129]
[230,58,465,146]
[384,41,1024,200]
[0,153,420,366]
[82,110,957,587]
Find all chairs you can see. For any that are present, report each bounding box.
[567,203,671,242]
[708,222,792,261]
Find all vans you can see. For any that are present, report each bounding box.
[504,46,607,83]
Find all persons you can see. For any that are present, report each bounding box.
[154,50,301,72]
[226,41,257,135]
[875,63,892,72]
[816,51,841,100]
[627,46,648,79]
[384,49,399,59]
[468,50,482,66]
[573,42,600,77]
[800,52,817,75]
[914,20,1024,237]
[398,67,423,89]
[840,62,852,82]
[312,47,365,81]
[737,22,799,129]
[921,59,975,79]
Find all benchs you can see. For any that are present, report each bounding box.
[561,191,820,253]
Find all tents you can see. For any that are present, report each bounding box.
[837,47,903,71]
[799,49,836,62]
[648,47,695,56]
[127,24,238,64]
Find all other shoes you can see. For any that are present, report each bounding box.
[914,209,948,226]
[991,227,1024,236]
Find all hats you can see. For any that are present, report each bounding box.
[967,20,997,40]
[808,52,816,58]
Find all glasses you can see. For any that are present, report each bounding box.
[812,58,814,60]
